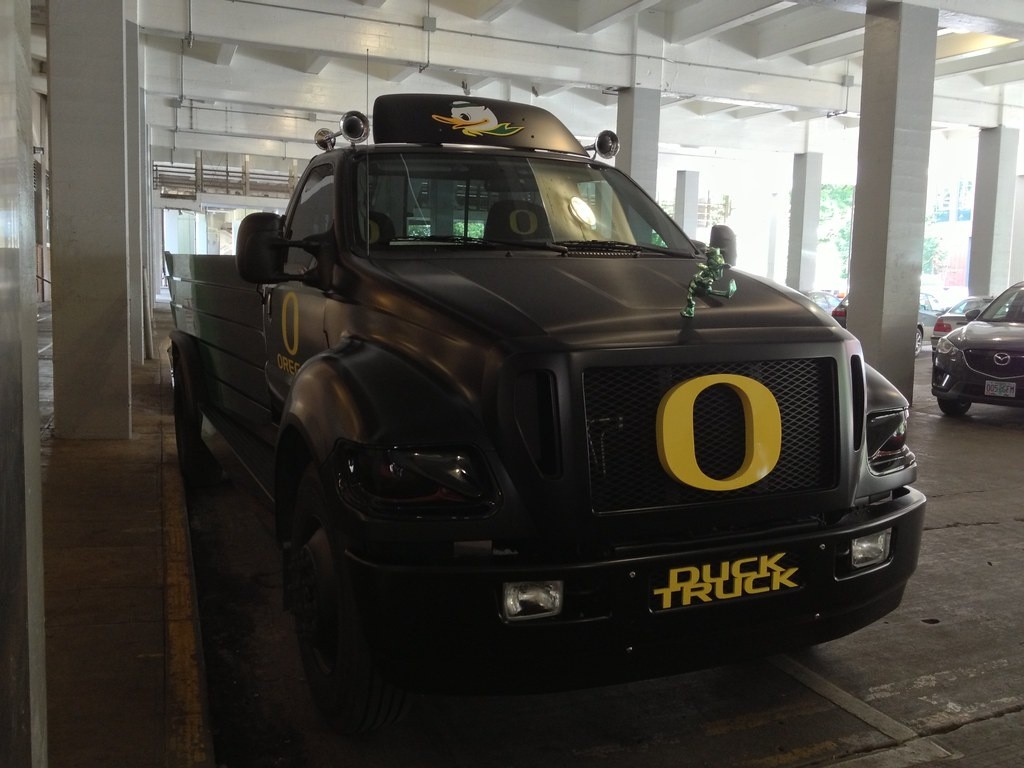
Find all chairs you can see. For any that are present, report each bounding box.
[484,198,556,240]
[365,211,396,240]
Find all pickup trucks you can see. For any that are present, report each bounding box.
[163,93,928,740]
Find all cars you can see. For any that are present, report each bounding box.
[929,278,1024,416]
[801,291,941,358]
[931,295,1012,349]
